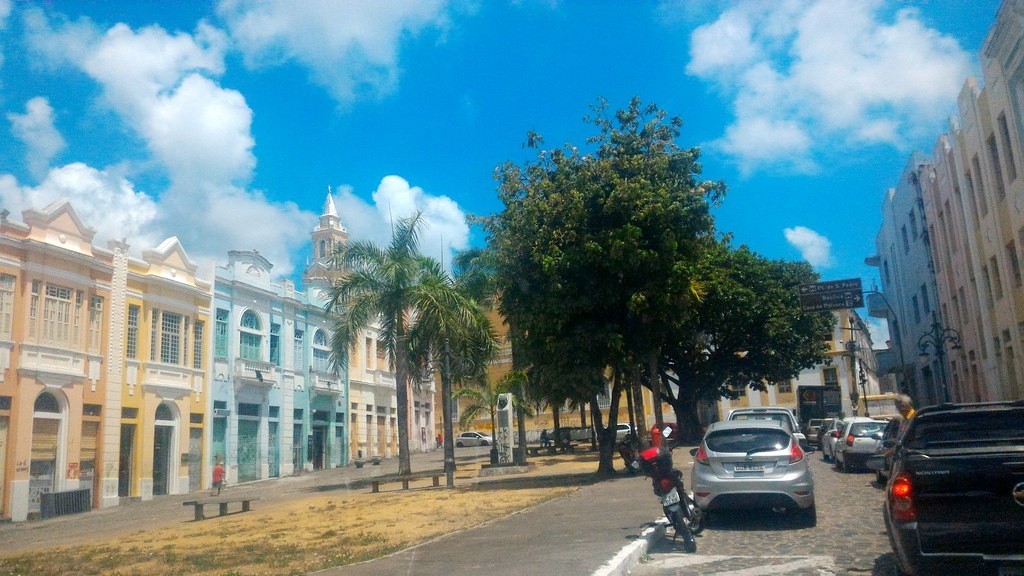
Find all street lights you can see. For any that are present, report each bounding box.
[917,310,964,404]
[421,337,477,472]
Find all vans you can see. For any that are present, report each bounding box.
[830,420,890,473]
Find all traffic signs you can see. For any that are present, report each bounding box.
[799,277,865,313]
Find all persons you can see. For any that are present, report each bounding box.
[437,433,442,448]
[540,428,552,448]
[210,462,226,496]
[872,391,916,459]
[556,427,571,451]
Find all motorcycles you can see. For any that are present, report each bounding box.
[627,425,706,554]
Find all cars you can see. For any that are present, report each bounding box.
[456,432,497,448]
[615,423,638,440]
[871,415,910,484]
[866,401,1023,576]
[652,423,678,437]
[821,416,874,464]
[727,406,799,433]
[801,419,823,444]
[688,420,814,515]
[815,418,838,450]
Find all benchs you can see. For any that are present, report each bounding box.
[183,496,261,521]
[364,468,445,493]
[525,438,594,457]
